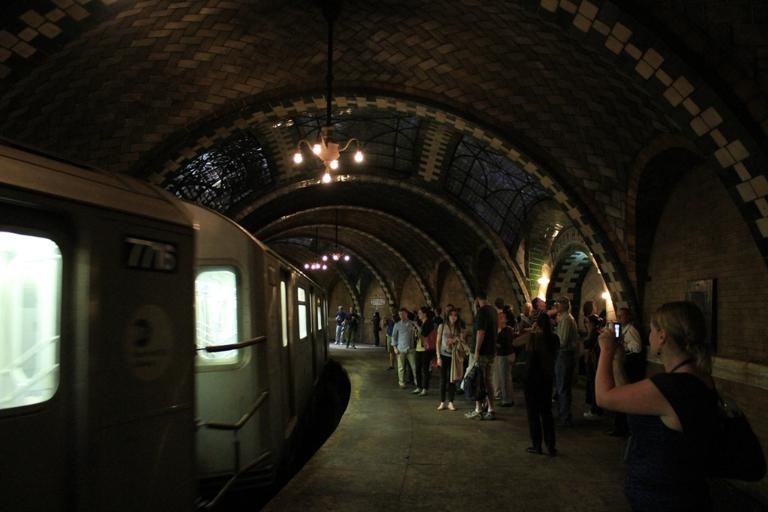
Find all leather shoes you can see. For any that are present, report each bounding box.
[418,391,427,396]
[411,388,420,393]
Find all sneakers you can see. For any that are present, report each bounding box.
[447,404,455,411]
[437,403,446,411]
[465,408,483,420]
[484,412,496,420]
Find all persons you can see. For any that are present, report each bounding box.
[593,301,722,509]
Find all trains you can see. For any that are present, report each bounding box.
[0,135,328,511]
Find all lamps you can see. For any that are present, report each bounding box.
[303,226,328,271]
[293,0,364,186]
[321,209,350,263]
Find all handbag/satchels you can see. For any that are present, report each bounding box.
[421,330,436,350]
[709,416,767,481]
[463,365,487,400]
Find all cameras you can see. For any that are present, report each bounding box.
[605,321,622,340]
